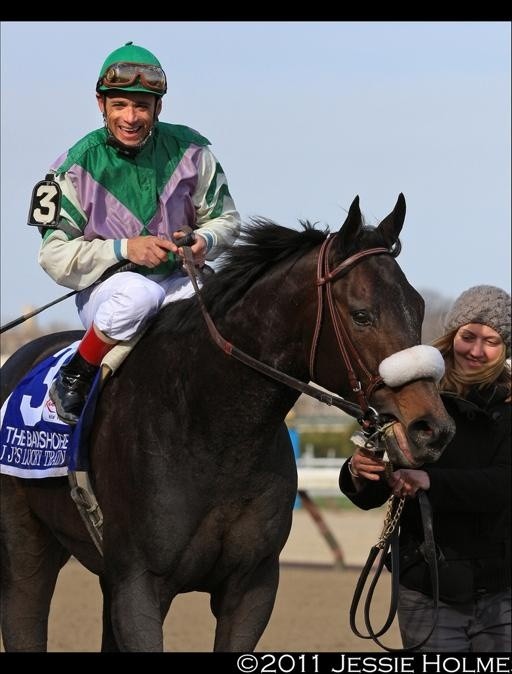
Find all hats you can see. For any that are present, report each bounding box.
[443,285,512,359]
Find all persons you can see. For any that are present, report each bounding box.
[338,284,512,654]
[26,41,242,427]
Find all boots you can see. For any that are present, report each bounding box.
[49,349,100,425]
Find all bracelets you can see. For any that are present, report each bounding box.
[347,457,360,478]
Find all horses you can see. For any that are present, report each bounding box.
[0,192,457,654]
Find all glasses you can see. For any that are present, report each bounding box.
[96,61,167,93]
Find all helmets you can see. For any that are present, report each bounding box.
[99,41,164,97]
